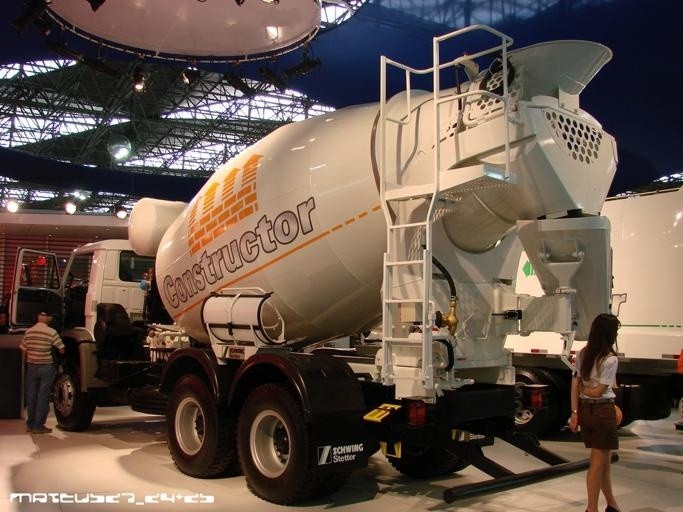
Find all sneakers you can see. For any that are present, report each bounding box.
[32,424,52,434]
[27,426,32,432]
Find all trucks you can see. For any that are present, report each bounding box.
[507,186,683,437]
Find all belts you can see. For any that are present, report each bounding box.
[580,398,615,404]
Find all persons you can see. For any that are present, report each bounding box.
[18,311,65,433]
[569,313,622,512]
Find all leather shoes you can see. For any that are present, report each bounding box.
[604,504,621,512]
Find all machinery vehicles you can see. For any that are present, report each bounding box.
[11,26,617,507]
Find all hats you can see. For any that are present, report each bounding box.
[39,304,57,316]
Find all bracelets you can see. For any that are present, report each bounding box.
[571,409,578,414]
